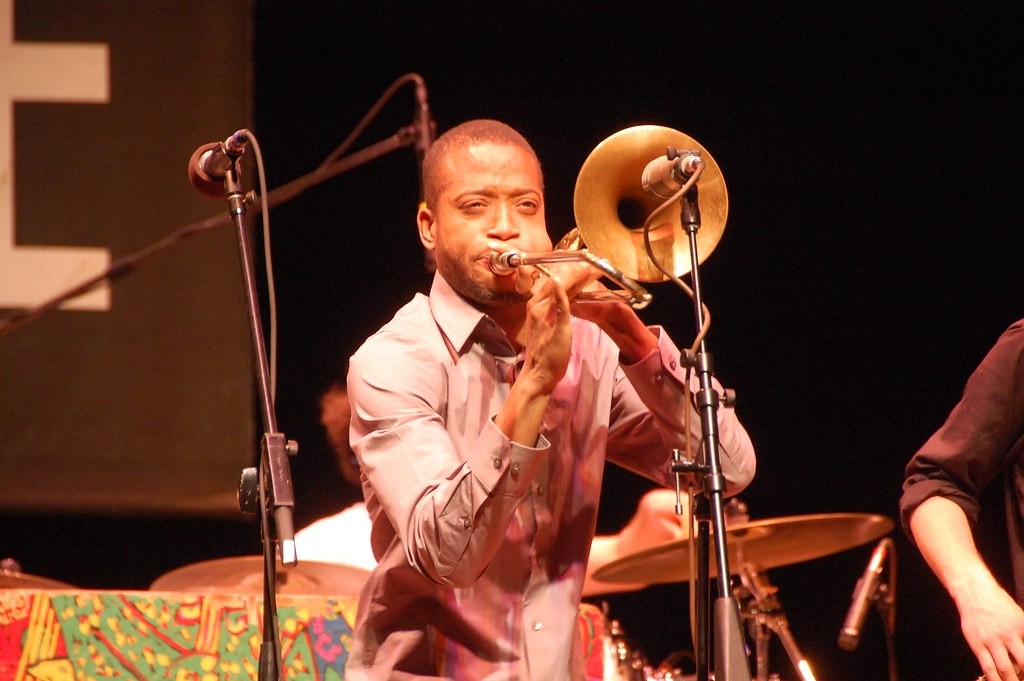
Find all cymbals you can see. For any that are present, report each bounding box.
[590,510,896,584]
[0,568,80,589]
[148,556,372,594]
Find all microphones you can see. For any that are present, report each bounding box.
[188,129,248,199]
[641,155,705,202]
[838,538,889,649]
[415,85,430,158]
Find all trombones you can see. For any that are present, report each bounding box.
[488,124,728,310]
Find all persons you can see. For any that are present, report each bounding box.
[278,121,756,681]
[900,318,1024,681]
